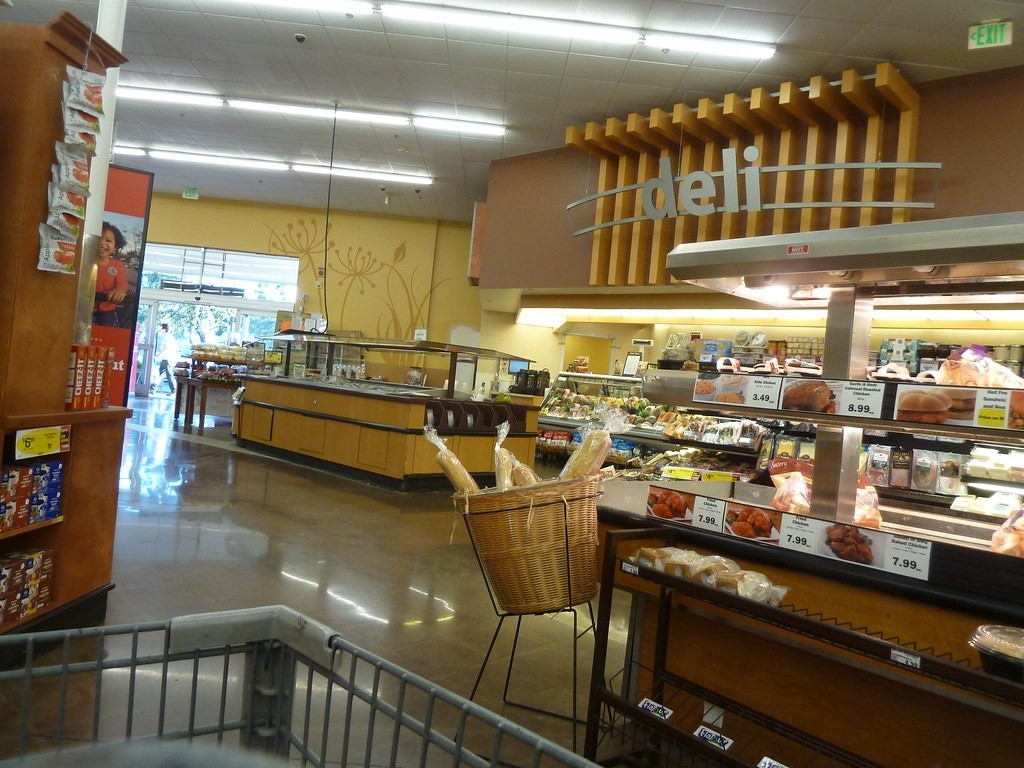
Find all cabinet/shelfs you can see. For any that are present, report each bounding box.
[0,425,75,631]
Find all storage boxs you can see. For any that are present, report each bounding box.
[15,425,70,460]
[880,334,919,377]
[0,461,63,530]
[0,549,55,626]
[698,338,732,363]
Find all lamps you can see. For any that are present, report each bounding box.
[117,85,507,137]
[114,145,435,186]
[238,0,777,60]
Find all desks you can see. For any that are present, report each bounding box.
[173,377,240,437]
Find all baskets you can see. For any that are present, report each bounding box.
[450,473,605,615]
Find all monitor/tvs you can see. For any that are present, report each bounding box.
[507,359,530,375]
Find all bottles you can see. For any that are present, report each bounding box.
[477,383,486,401]
[332,363,366,379]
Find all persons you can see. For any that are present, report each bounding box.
[93,221,128,327]
[150,324,175,394]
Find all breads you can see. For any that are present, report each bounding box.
[436,429,613,493]
[634,546,743,596]
[896,387,976,424]
[782,380,831,412]
